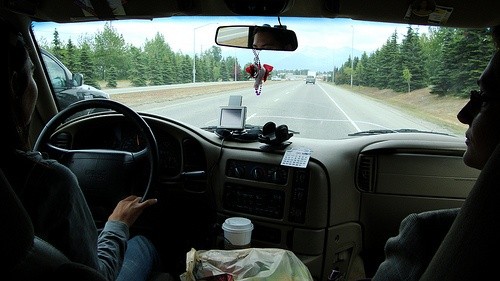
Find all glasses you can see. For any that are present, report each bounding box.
[469,89,500,108]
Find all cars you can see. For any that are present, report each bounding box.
[305,76,316,84]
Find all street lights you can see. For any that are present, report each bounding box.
[192,21,220,84]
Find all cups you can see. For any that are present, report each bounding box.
[222,217,254,249]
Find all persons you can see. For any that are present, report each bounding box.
[371,25,500,280]
[252,26,292,49]
[0,8,161,281]
